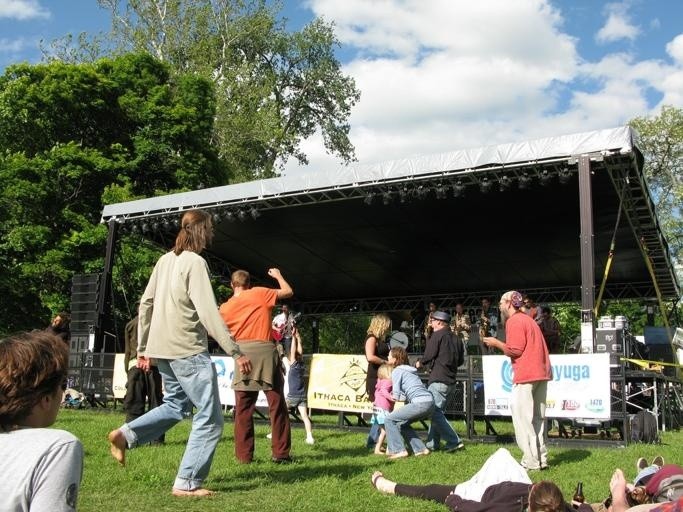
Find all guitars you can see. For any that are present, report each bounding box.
[272,313,301,340]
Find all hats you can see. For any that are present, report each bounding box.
[429,310,453,324]
[499,290,525,308]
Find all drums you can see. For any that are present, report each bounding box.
[414,329,422,338]
[387,330,409,351]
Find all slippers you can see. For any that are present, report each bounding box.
[370,471,389,497]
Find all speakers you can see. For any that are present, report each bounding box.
[69,272,103,332]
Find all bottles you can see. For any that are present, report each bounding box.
[572,482,584,504]
[463,309,482,324]
[625,381,637,395]
[446,310,456,321]
[489,308,501,322]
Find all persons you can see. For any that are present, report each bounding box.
[266,326,314,444]
[609,468,683,512]
[364,291,560,470]
[45,313,68,343]
[272,303,296,354]
[125,303,165,446]
[0,329,84,512]
[370,448,594,512]
[109,210,253,497]
[219,268,294,464]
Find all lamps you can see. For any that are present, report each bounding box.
[362,164,574,209]
[130,205,260,235]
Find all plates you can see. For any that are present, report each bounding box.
[415,373,427,376]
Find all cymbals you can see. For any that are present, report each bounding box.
[406,320,419,327]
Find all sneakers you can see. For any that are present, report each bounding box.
[636,457,649,474]
[414,447,431,456]
[651,455,665,471]
[387,448,409,461]
[446,441,465,453]
[271,456,299,465]
